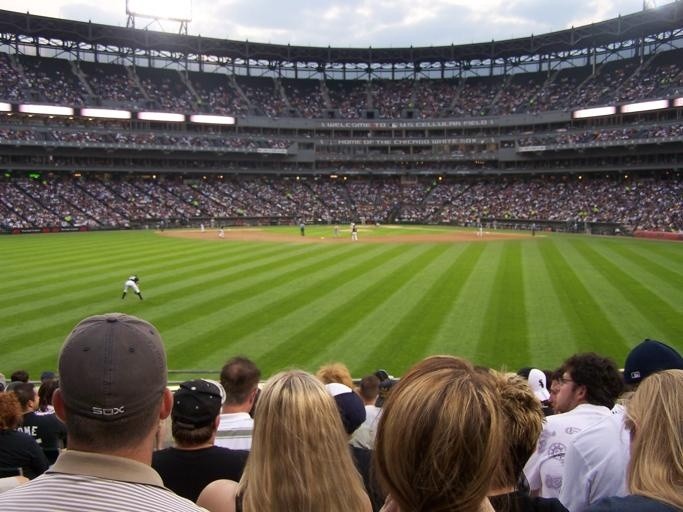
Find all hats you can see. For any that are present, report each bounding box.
[171,378,227,431]
[59,312,167,421]
[325,383,366,429]
[624,339,683,384]
[517,368,551,402]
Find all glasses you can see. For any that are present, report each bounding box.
[559,378,573,386]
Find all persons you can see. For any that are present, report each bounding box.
[350,223,358,241]
[218,226,225,239]
[300,222,305,236]
[477,223,484,237]
[121,274,144,301]
[334,223,338,237]
[0,311,683,512]
[1,54,683,234]
[530,223,536,237]
